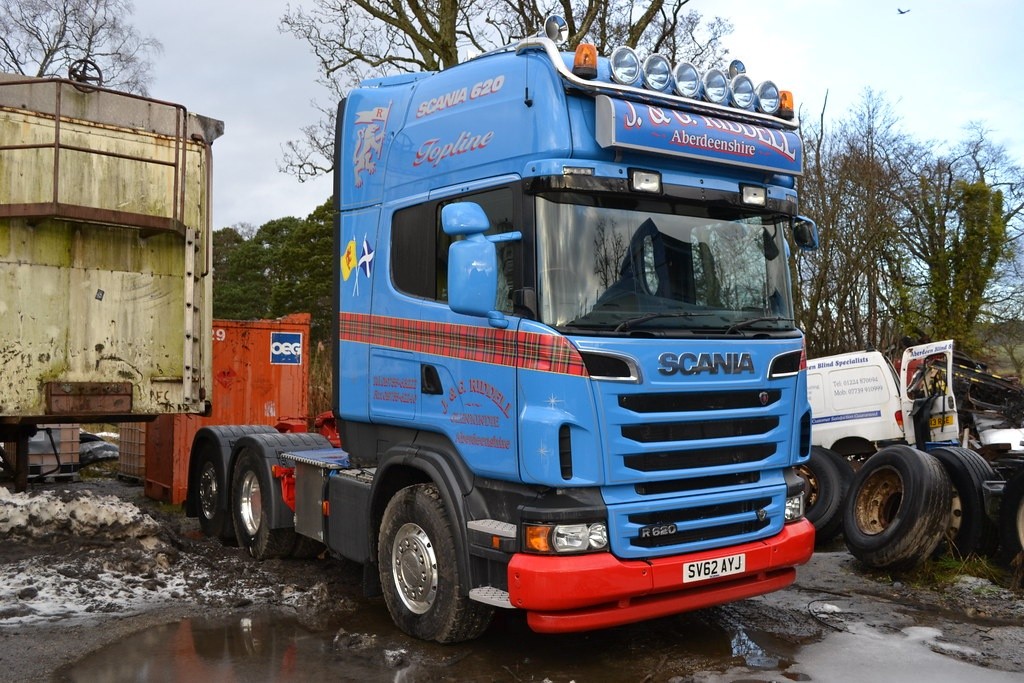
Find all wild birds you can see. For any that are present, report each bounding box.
[898,8,911,14]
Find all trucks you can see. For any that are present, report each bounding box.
[805,349,906,458]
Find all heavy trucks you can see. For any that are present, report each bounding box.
[184,13,816,644]
